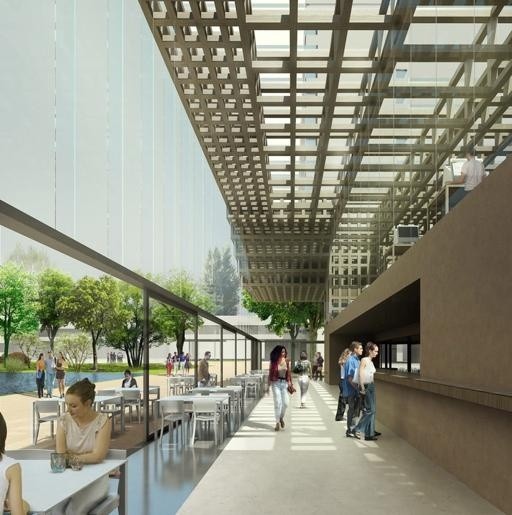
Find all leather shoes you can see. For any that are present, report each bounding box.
[374,431,381,436]
[352,429,361,440]
[365,436,377,440]
[275,423,279,431]
[280,420,284,428]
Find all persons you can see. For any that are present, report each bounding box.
[55,378,111,515]
[445,147,487,193]
[122,370,137,388]
[298,351,312,408]
[36,350,65,398]
[268,345,294,431]
[166,352,190,376]
[336,341,382,441]
[198,352,211,395]
[107,352,123,363]
[0,412,30,515]
[312,352,323,381]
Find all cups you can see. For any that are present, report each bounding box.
[51,453,66,473]
[68,450,85,471]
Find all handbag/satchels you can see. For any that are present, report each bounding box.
[293,365,303,373]
[287,384,296,395]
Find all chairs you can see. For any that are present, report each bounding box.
[87,449,128,515]
[4,370,269,460]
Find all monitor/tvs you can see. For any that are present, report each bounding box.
[450,159,467,175]
[398,224,419,240]
[442,167,453,184]
